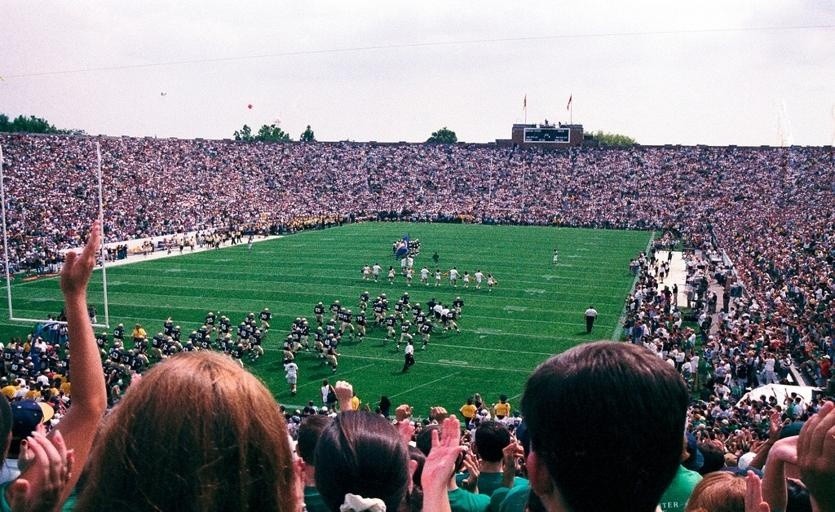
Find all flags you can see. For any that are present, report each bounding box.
[524,95,526,108]
[567,94,572,110]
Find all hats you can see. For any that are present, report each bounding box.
[738,452,757,474]
[11,400,54,436]
